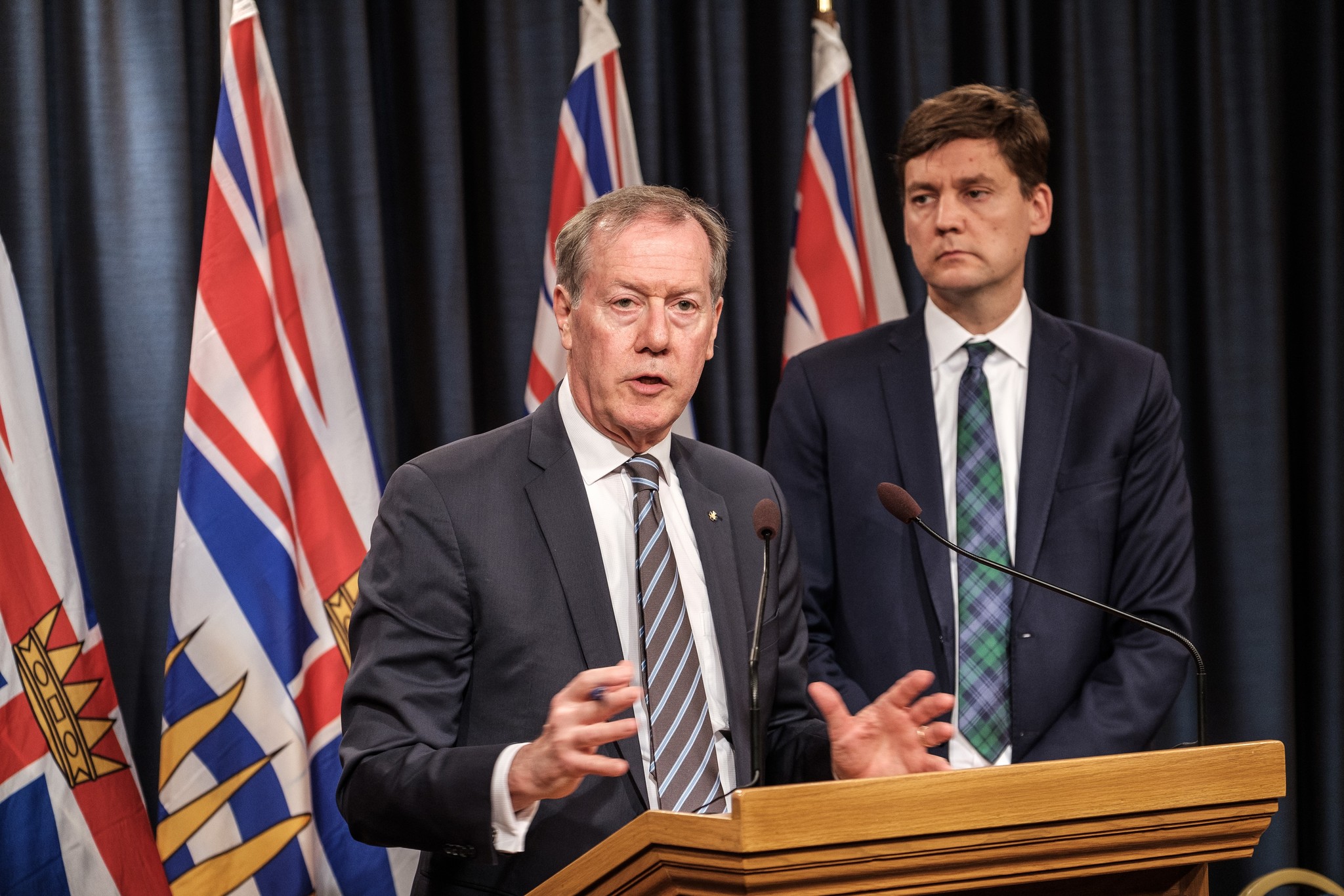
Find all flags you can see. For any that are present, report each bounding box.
[153,0,421,896]
[0,239,171,896]
[523,0,700,448]
[778,16,909,380]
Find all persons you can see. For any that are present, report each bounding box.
[336,186,955,896]
[767,83,1196,771]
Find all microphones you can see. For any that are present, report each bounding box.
[749,498,781,785]
[878,482,1207,745]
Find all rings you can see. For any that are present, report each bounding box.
[917,725,935,748]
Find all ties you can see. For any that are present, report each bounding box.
[956,341,1015,768]
[623,451,731,817]
